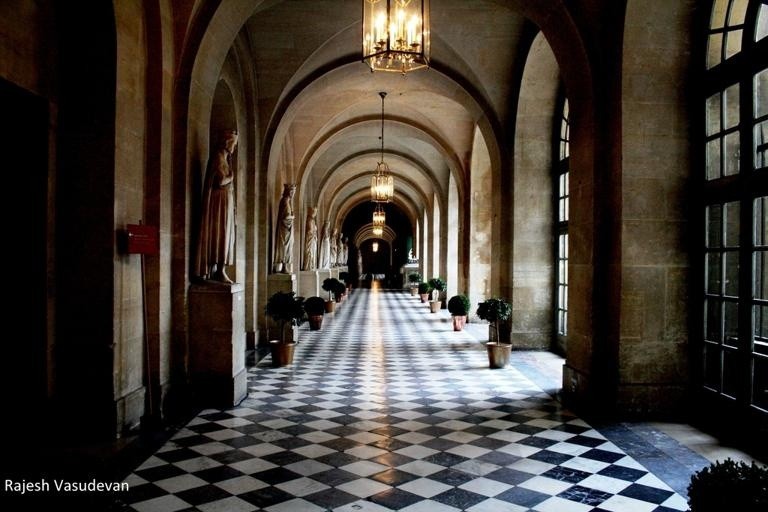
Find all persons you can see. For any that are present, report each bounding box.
[301,205,318,272]
[191,128,240,285]
[316,220,349,271]
[272,182,297,276]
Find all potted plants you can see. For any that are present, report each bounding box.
[263,291,305,367]
[321,272,445,314]
[305,297,326,332]
[475,298,512,370]
[447,295,468,332]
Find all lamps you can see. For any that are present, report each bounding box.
[373,236,380,252]
[373,225,384,235]
[372,203,387,224]
[361,1,430,75]
[371,92,394,204]
